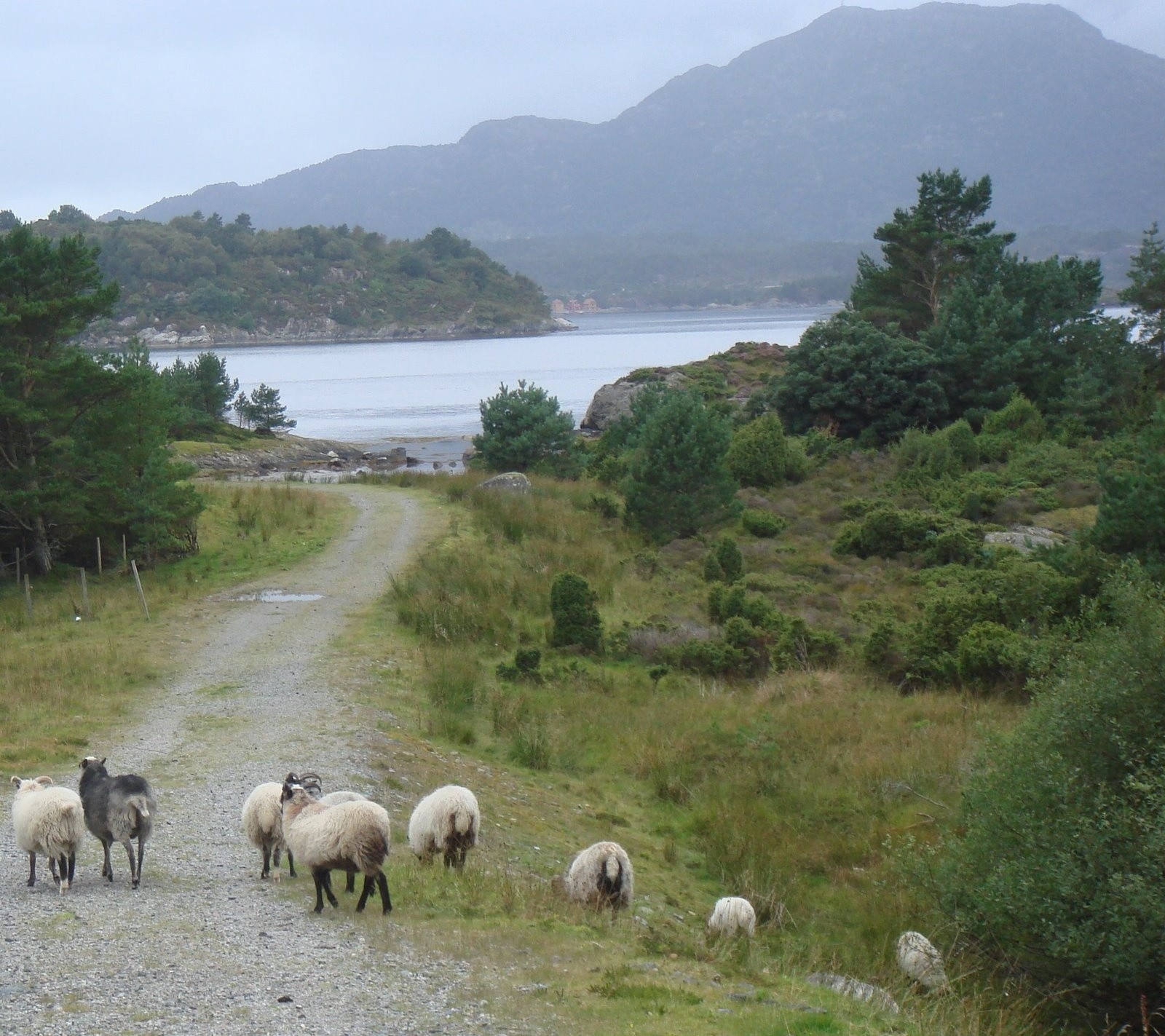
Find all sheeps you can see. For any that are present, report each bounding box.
[9,775,85,896]
[239,773,393,915]
[706,897,756,938]
[564,843,635,922]
[77,755,156,890]
[897,931,949,997]
[409,786,481,875]
